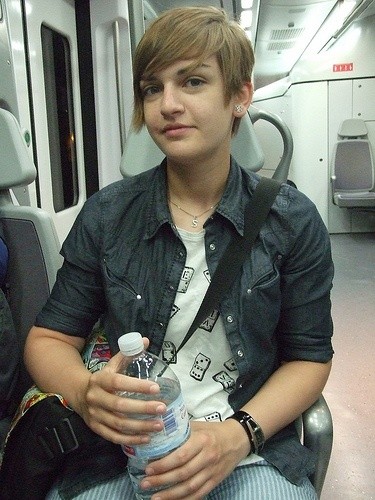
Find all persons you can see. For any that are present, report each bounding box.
[0,286,20,451]
[23,6,335,500]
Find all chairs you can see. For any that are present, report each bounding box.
[0,103,375,500]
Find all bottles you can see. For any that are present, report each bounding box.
[113,333,190,500]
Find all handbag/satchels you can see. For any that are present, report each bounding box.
[0,328,128,500]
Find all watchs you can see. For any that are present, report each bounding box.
[232,411,265,455]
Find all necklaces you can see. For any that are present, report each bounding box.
[167,197,221,228]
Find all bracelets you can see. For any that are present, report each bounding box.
[225,417,253,456]
